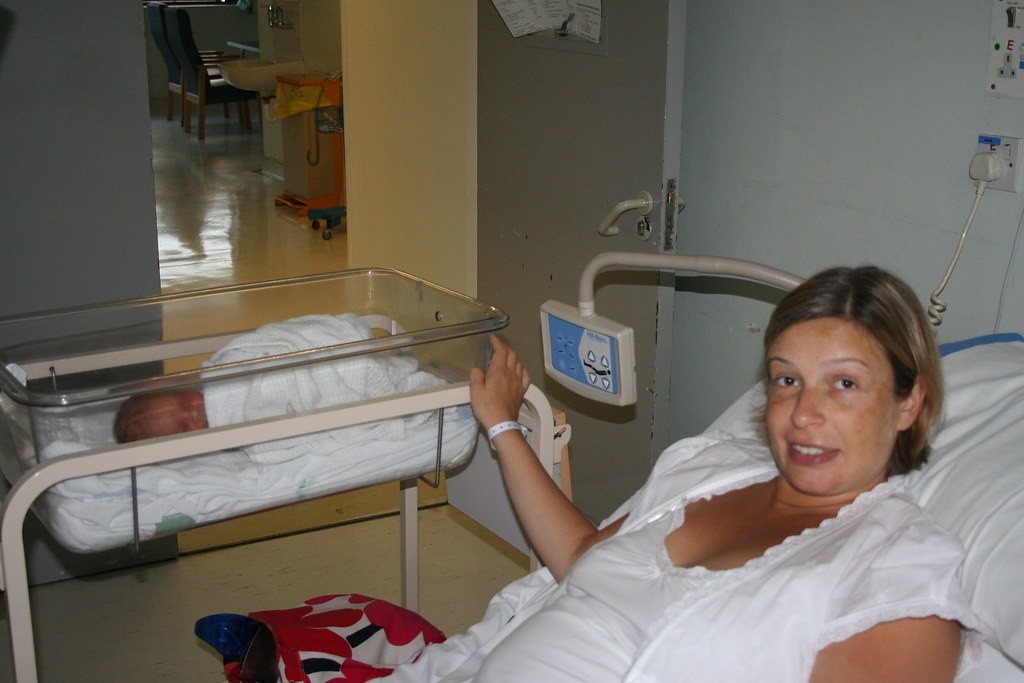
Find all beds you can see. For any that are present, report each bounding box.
[367,332,1024,683]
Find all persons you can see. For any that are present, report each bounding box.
[114,311,453,464]
[471,264,979,683]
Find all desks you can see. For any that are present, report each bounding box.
[225,39,260,60]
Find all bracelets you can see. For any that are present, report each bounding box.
[487,421,532,450]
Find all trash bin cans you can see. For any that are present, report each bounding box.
[271,71,343,217]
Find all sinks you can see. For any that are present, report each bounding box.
[218,58,304,93]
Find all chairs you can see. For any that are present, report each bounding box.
[162,7,263,141]
[147,1,243,128]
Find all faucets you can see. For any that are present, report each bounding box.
[258,3,285,30]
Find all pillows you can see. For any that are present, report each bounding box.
[906,339,1024,669]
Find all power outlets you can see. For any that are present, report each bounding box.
[976,133,1024,194]
[984,0,1024,100]
